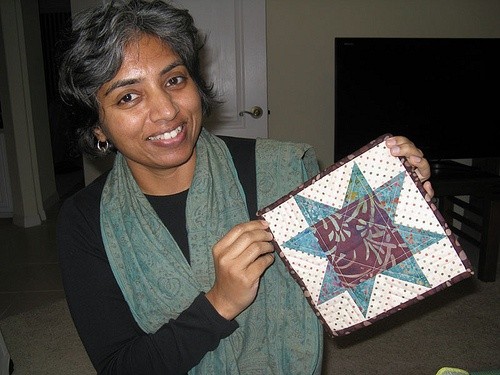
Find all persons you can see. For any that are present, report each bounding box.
[54,2,436,375]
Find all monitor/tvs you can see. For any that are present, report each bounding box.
[334,38,500,167]
